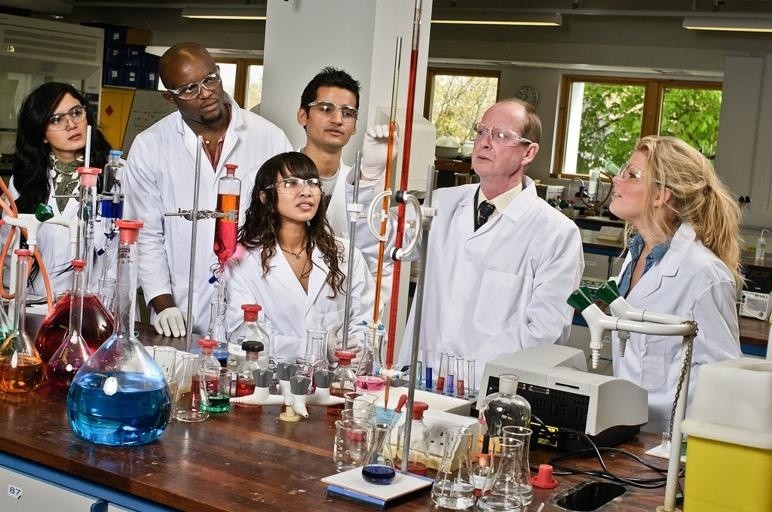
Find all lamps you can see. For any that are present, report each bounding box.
[181,7,267,20]
[681,16,772,32]
[430,12,563,27]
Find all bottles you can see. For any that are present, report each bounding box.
[586,169,598,201]
[573,186,588,206]
[547,196,572,212]
[461,141,474,157]
[0,150,330,447]
[755,237,767,262]
[326,331,534,512]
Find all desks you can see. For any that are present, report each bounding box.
[736,302,772,357]
[0,320,686,512]
[579,229,629,282]
[739,248,772,293]
[433,156,472,173]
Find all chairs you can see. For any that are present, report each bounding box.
[453,172,479,187]
[433,169,439,189]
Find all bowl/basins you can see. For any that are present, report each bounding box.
[435,146,458,161]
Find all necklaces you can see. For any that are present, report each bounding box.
[282,247,307,259]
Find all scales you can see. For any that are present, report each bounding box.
[321,464,436,511]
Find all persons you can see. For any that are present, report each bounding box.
[610,136,746,440]
[345,96,586,391]
[223,151,384,366]
[0,81,141,322]
[122,41,295,340]
[237,66,398,316]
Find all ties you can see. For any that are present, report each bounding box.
[474,202,496,230]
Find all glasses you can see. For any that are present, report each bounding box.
[308,100,358,124]
[47,105,87,130]
[166,68,222,100]
[617,162,651,185]
[469,121,534,146]
[265,178,322,194]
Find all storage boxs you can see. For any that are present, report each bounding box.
[80,22,161,91]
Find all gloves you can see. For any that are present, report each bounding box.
[327,322,360,363]
[361,126,401,180]
[153,307,194,338]
[351,343,371,374]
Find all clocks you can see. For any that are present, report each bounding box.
[515,85,539,106]
